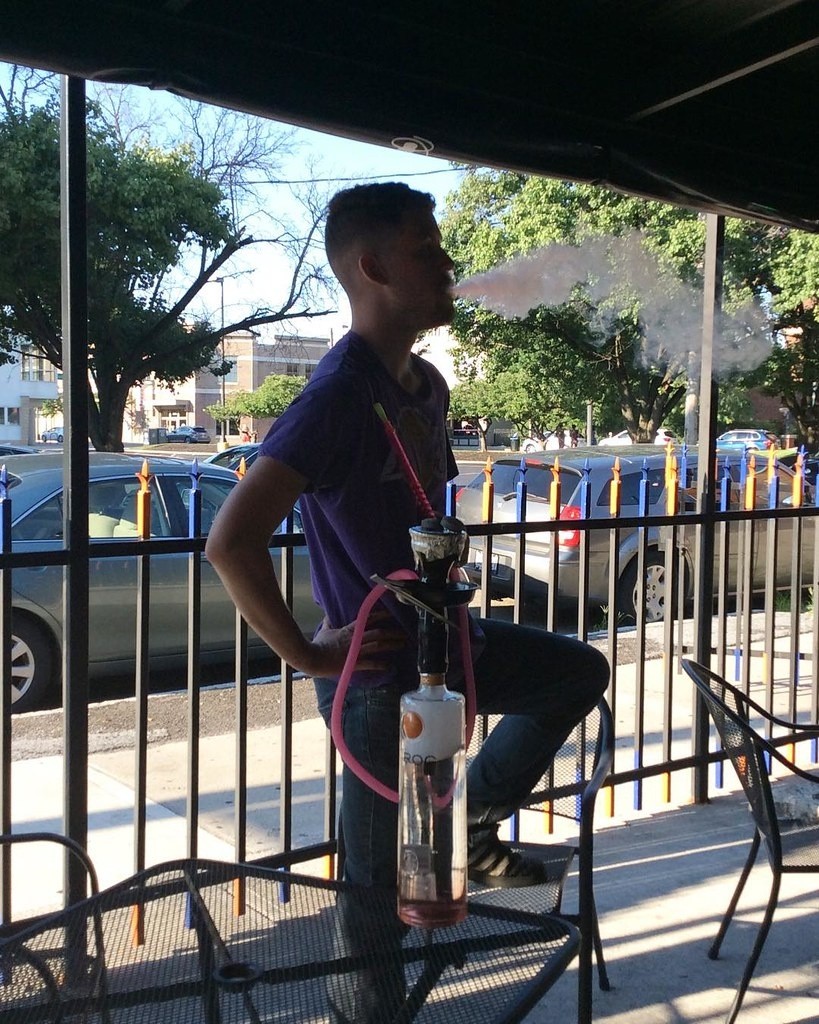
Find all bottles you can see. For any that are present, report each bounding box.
[395,669,469,928]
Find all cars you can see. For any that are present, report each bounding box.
[714,428,782,455]
[0,452,331,712]
[0,442,41,458]
[41,426,64,443]
[598,427,684,446]
[453,443,819,626]
[520,429,586,455]
[197,442,304,515]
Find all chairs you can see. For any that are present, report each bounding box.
[88,482,158,537]
[681,659,819,1024]
[338,697,615,1024]
[0,833,110,1024]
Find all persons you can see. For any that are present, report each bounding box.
[205,183,609,1024]
[556,423,564,449]
[569,425,578,448]
[591,425,597,445]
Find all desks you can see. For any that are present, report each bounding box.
[0,858,582,1024]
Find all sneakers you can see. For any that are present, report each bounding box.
[467,822,549,887]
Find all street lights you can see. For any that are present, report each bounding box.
[215,275,231,453]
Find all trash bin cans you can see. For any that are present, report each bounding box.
[148,428,168,445]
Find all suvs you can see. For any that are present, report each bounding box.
[167,426,212,445]
